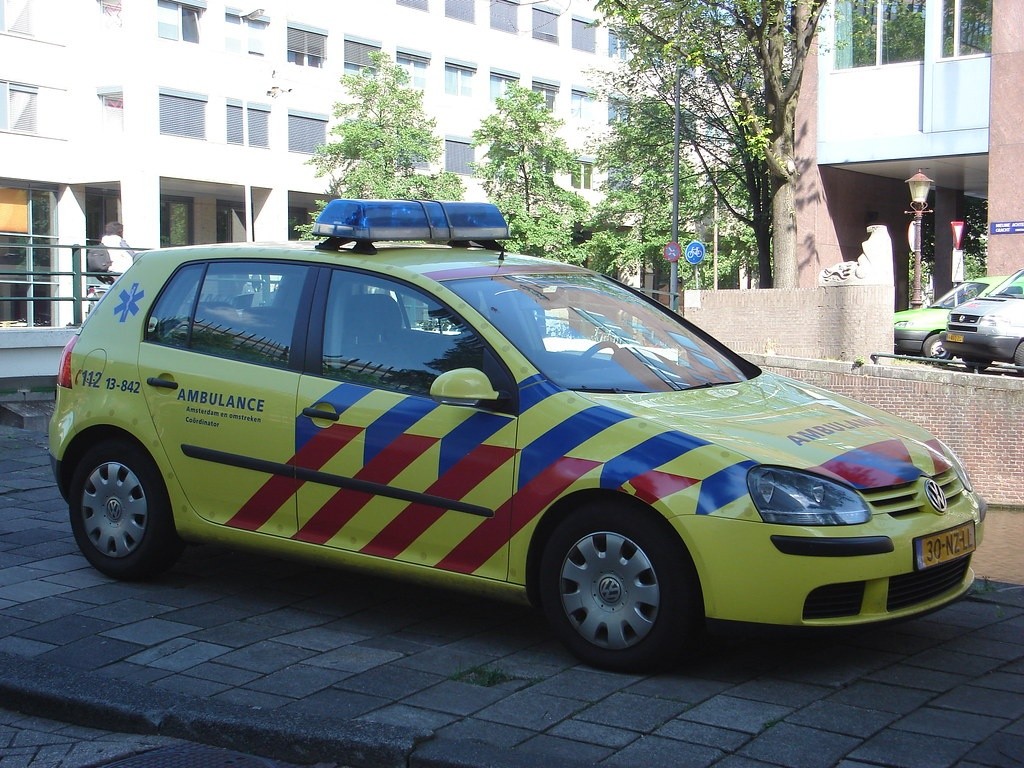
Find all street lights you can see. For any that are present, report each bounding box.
[904,169,935,310]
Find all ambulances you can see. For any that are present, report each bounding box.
[47,198,990,674]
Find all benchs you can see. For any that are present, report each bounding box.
[187,301,293,365]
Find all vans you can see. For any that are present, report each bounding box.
[938,265,1024,381]
[894,276,1024,366]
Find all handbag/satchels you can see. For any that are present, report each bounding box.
[86,248,112,272]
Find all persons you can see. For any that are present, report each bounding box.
[101,222,137,285]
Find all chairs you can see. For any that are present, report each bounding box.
[341,294,416,374]
[428,299,485,373]
